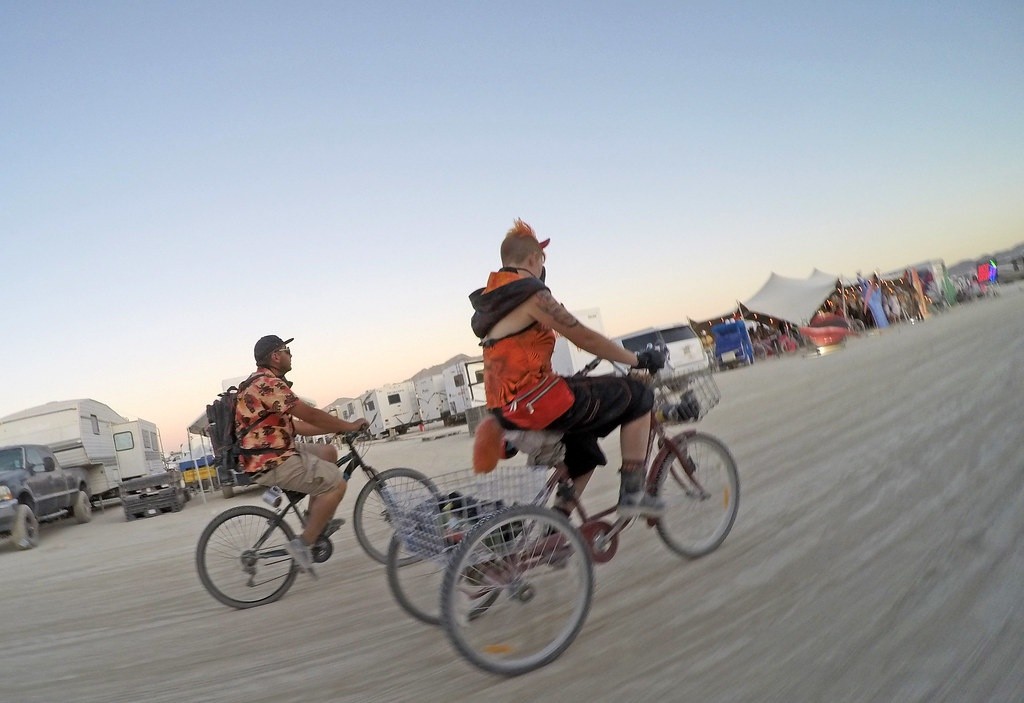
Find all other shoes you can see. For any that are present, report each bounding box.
[545,540,573,566]
[615,470,669,515]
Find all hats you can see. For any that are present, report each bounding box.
[254,335,294,361]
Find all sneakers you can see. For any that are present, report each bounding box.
[301,512,344,537]
[284,541,319,581]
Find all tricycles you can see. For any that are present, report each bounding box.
[383,329,746,678]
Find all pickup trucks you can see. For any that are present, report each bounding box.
[0,444,92,551]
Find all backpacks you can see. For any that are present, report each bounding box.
[207,373,287,471]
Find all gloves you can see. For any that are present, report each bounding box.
[636,349,665,373]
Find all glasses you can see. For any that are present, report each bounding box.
[276,348,291,355]
[538,251,547,262]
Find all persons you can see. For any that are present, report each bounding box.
[469,218,666,563]
[235,335,370,580]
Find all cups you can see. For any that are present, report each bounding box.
[262,485,283,508]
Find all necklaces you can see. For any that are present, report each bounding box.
[517,268,536,277]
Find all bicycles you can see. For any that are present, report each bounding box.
[195,413,439,609]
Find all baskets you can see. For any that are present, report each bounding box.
[644,364,720,426]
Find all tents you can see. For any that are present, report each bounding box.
[734,267,842,358]
